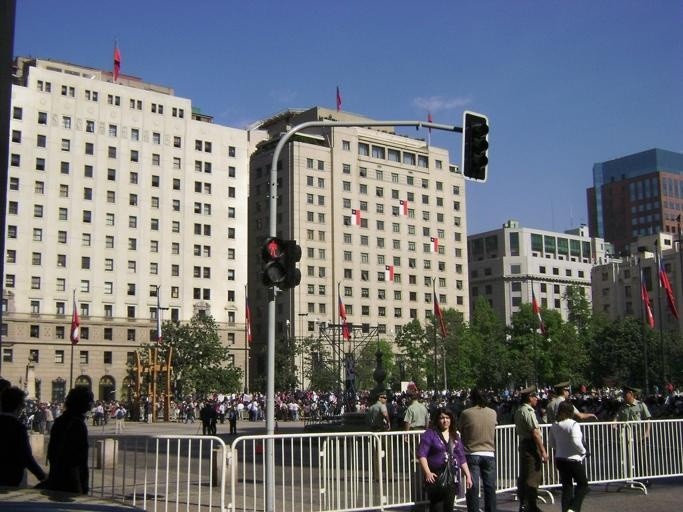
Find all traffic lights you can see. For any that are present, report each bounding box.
[261,237,302,288]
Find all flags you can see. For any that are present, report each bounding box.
[337,86,341,112]
[658,253,680,320]
[428,114,432,134]
[339,295,350,341]
[245,301,253,343]
[435,293,446,336]
[114,48,120,81]
[532,288,545,334]
[399,200,407,215]
[70,301,80,344]
[385,265,393,281]
[430,237,438,252]
[352,209,360,225]
[640,269,655,329]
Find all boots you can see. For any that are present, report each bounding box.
[519,499,542,512]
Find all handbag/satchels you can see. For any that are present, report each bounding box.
[425,464,460,500]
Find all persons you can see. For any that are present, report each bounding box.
[0,387,48,487]
[514,385,548,512]
[458,387,497,512]
[612,385,652,487]
[548,400,588,512]
[45,386,94,495]
[369,390,392,482]
[403,390,429,481]
[545,382,598,485]
[417,408,473,512]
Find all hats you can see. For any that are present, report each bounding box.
[377,390,388,398]
[523,384,539,399]
[621,384,640,394]
[554,380,572,393]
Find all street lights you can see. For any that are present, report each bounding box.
[460,110,490,184]
